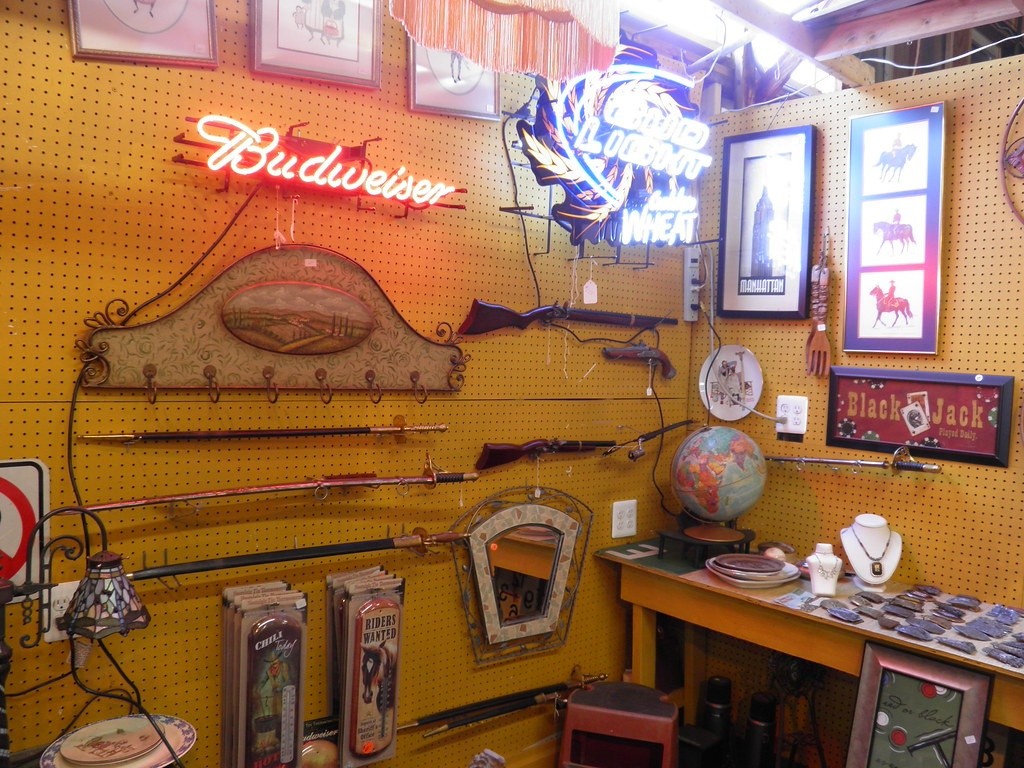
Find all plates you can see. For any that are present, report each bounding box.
[40,713,197,768]
[705,553,802,589]
[699,344,764,422]
[58,715,166,765]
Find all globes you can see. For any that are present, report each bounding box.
[670,426,768,543]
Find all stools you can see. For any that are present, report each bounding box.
[559,682,680,768]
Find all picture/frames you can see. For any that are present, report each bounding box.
[840,642,997,768]
[722,125,815,320]
[841,99,947,353]
[821,365,1016,467]
[250,0,383,88]
[70,0,221,66]
[406,37,502,121]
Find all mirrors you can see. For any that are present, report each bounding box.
[450,486,593,665]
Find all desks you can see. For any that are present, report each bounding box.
[600,534,1024,733]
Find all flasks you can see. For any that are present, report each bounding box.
[703,674,732,737]
[741,692,777,768]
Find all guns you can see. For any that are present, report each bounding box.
[475,436,617,470]
[457,297,680,335]
[602,339,677,379]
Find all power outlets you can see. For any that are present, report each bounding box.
[774,393,810,435]
[614,499,638,536]
